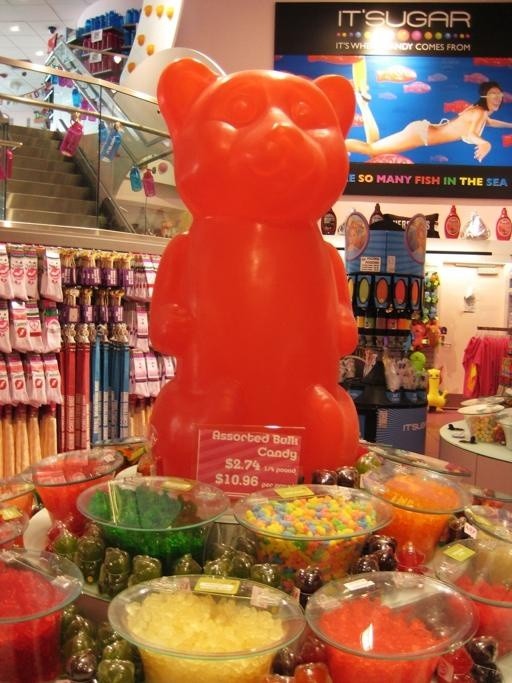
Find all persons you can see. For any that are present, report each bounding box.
[344,81,510,163]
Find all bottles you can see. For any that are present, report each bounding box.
[76,7,140,34]
[81,29,136,92]
[495,207,512,240]
[98,120,124,164]
[443,204,461,240]
[321,207,337,235]
[1,148,13,180]
[369,202,384,224]
[130,164,156,197]
[59,121,84,157]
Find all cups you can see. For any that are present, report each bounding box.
[107,574,306,682]
[19,448,124,531]
[362,465,465,554]
[368,443,472,477]
[1,546,84,683]
[77,476,230,568]
[305,570,478,683]
[458,396,512,449]
[432,536,512,656]
[464,505,512,543]
[233,483,395,567]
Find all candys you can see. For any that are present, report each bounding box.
[0,414,512,683]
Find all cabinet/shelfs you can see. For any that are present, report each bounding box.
[64,22,137,88]
